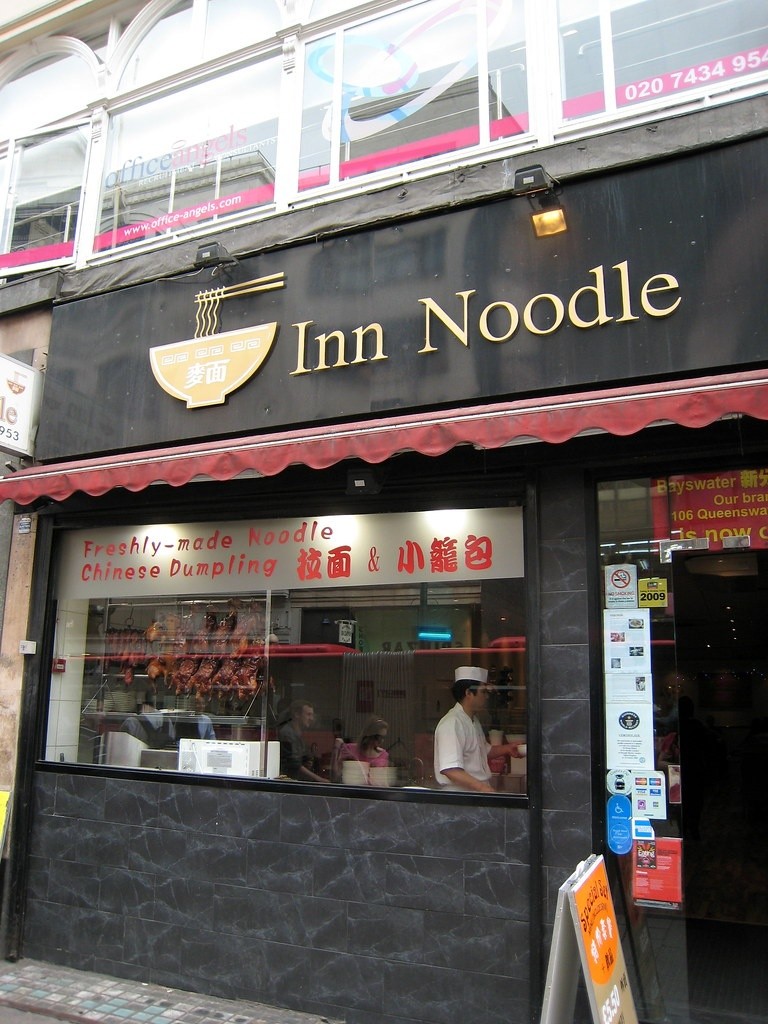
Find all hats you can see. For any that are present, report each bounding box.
[455,666,488,683]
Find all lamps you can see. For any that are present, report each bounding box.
[511,164,564,200]
[526,195,569,239]
[194,242,235,268]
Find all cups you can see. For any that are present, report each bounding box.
[488,729,504,746]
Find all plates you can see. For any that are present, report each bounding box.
[103,691,138,712]
[81,698,115,712]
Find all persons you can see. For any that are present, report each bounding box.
[706,715,722,735]
[337,716,389,785]
[174,693,216,740]
[119,678,176,749]
[739,716,768,745]
[679,696,709,838]
[434,667,524,792]
[278,700,330,783]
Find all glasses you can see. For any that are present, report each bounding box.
[372,733,386,741]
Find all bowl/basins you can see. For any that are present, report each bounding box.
[517,744,527,756]
[369,763,399,786]
[342,760,370,786]
[505,734,527,744]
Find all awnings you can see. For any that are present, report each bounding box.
[0,369,768,506]
